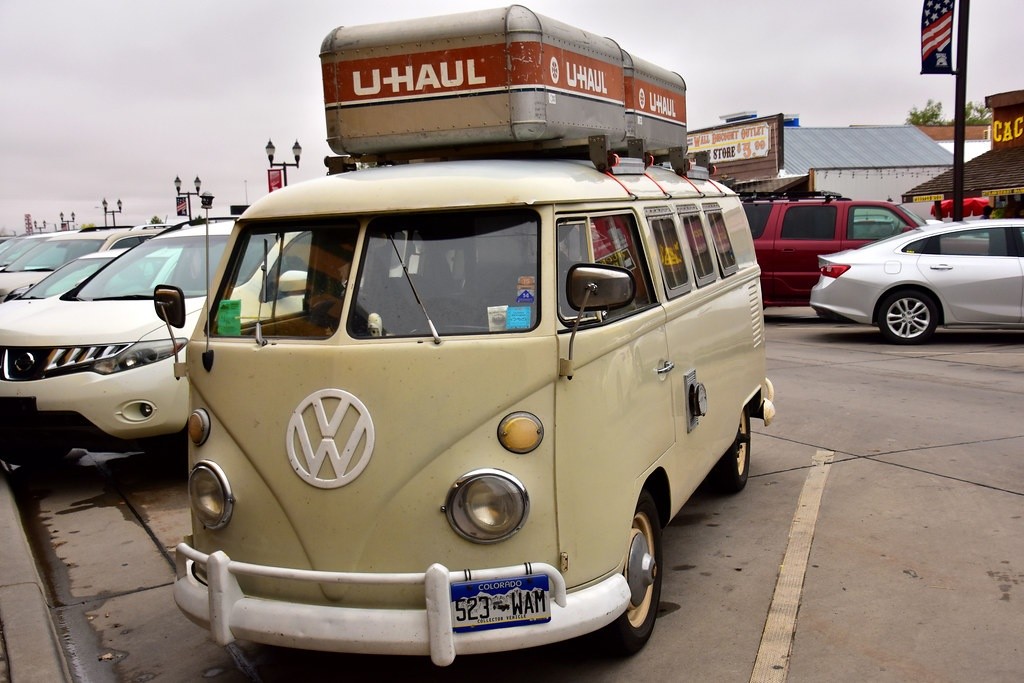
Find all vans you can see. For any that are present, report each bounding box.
[153,4,777,666]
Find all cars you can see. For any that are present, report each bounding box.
[0,244,185,310]
[809,219,1024,345]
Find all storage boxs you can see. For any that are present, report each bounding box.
[319,5,624,151]
[605,51,686,154]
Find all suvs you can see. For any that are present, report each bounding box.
[0,215,419,478]
[740,191,984,309]
[0,222,174,305]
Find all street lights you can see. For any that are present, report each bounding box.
[102,198,122,227]
[60,211,76,231]
[34,220,46,233]
[174,174,201,221]
[265,137,302,188]
